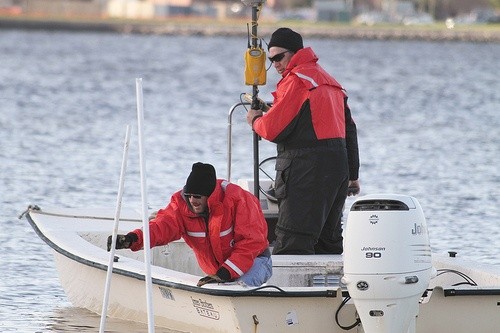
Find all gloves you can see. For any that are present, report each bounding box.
[107,234,135,251]
[197,267,231,287]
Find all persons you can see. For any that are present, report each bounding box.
[246,27,359,255]
[107,162,273,287]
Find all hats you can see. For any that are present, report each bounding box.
[183,162,216,196]
[268,28,304,52]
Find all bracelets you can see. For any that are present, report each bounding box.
[251,114,264,128]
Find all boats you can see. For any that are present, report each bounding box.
[18,101,500,333]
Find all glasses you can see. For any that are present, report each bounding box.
[187,194,202,199]
[268,50,291,62]
